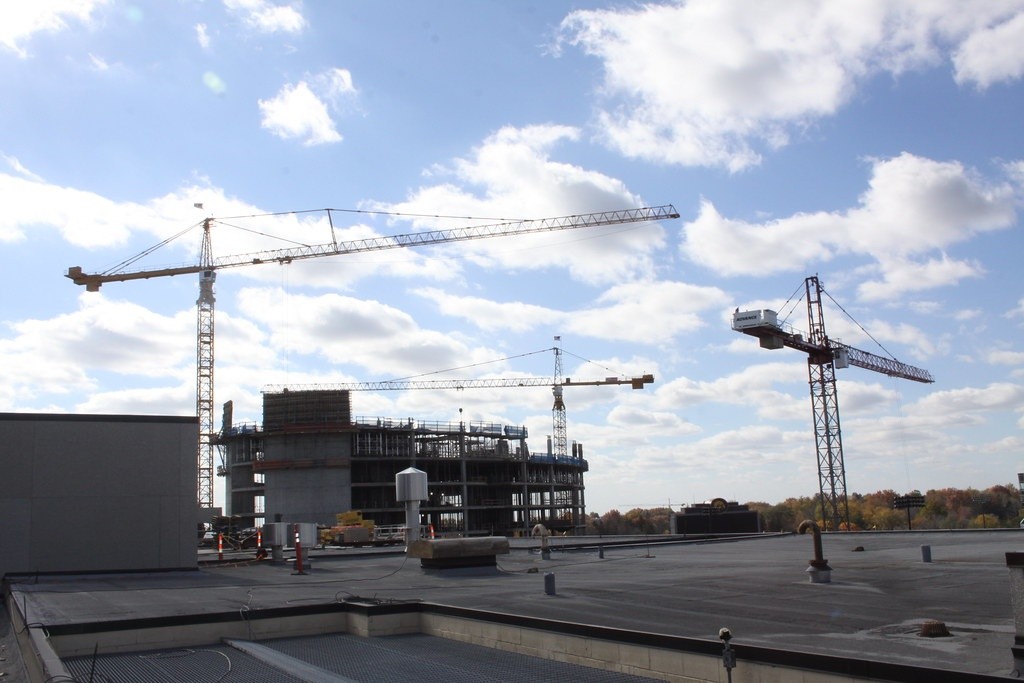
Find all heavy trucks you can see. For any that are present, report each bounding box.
[336,523,426,547]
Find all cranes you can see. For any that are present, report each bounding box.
[730,275,935,532]
[64,202,683,555]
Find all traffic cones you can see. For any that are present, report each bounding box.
[257,531,262,551]
[218,533,224,560]
[429,525,434,540]
